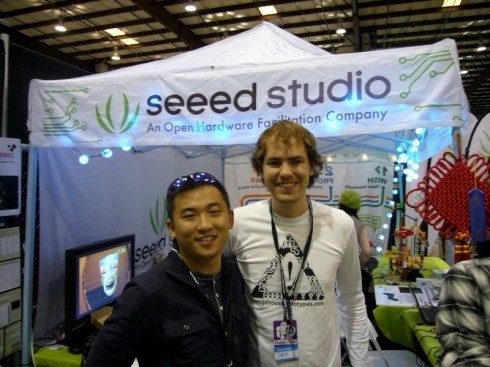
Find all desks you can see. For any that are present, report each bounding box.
[401,308,447,367]
[371,254,453,351]
[34,342,84,367]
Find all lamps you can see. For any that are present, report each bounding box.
[54,9,67,32]
[460,60,468,75]
[476,33,487,52]
[335,12,346,34]
[110,45,121,61]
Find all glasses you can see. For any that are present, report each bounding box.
[166,172,217,197]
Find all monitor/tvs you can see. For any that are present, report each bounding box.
[64,233,136,350]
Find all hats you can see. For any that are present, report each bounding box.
[339,190,362,209]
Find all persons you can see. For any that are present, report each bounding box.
[83,173,250,367]
[221,120,370,367]
[339,190,371,271]
[435,256,490,367]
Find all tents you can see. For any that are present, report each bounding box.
[23,18,471,367]
